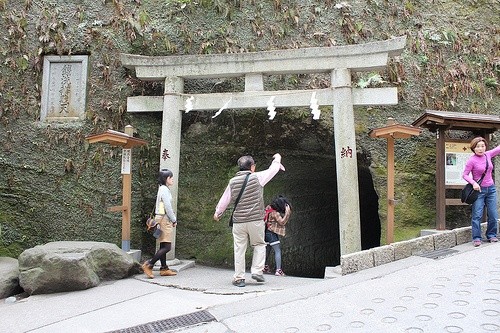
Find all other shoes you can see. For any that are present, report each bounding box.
[232,279,245,287]
[263,265,271,274]
[160,270,177,275]
[142,261,154,278]
[488,237,498,241]
[252,274,265,282]
[275,268,286,276]
[473,240,480,246]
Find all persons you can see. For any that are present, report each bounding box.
[142,169,179,278]
[214,153,285,287]
[263,195,292,275]
[462,137,500,247]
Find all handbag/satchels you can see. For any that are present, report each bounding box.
[144,218,163,239]
[229,216,233,227]
[461,180,483,205]
